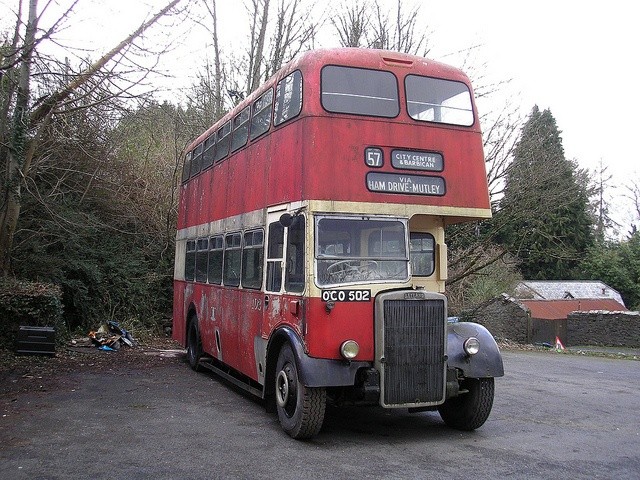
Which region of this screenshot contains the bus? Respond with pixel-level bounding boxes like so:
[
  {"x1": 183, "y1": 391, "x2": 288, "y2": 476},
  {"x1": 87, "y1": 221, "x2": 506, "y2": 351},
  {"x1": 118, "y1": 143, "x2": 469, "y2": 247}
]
[{"x1": 171, "y1": 46, "x2": 508, "y2": 442}]
